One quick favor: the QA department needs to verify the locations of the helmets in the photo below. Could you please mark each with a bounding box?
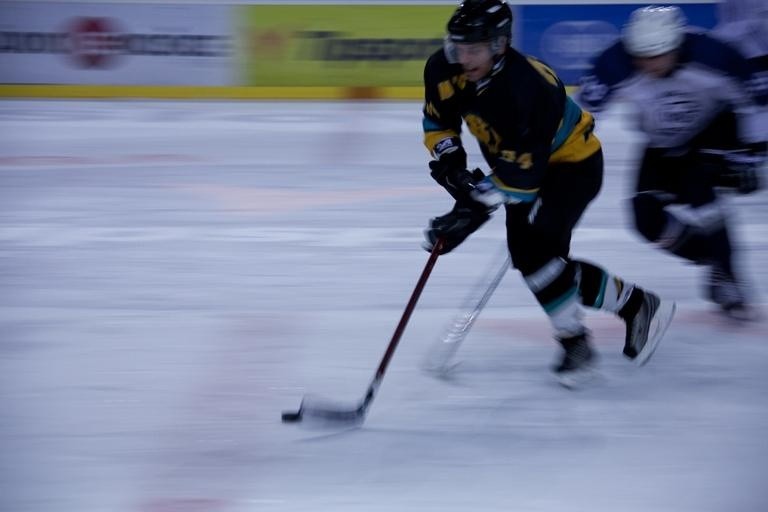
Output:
[619,6,685,58]
[447,0,513,44]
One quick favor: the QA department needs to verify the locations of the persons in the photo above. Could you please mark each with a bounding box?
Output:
[570,1,768,310]
[422,1,661,374]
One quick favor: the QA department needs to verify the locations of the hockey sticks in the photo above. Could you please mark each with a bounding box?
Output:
[430,256,512,379]
[297,238,441,430]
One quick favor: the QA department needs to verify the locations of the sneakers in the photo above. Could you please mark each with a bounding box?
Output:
[557,291,659,369]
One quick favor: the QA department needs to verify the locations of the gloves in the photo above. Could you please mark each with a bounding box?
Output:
[424,151,493,254]
